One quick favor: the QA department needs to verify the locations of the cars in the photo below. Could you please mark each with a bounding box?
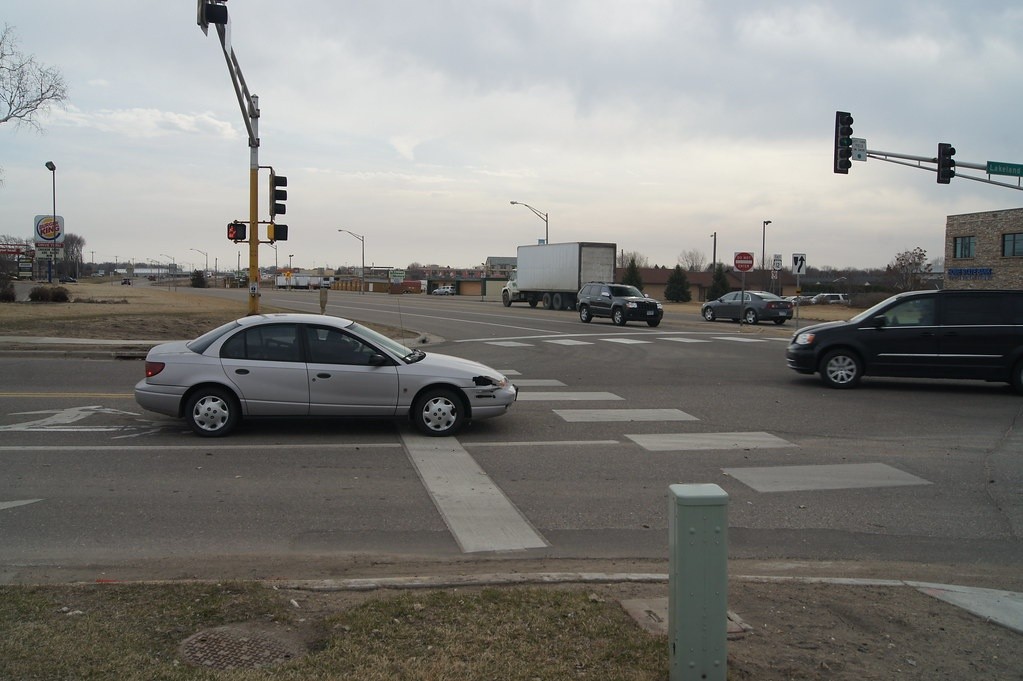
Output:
[780,294,854,308]
[121,278,131,285]
[58,276,77,283]
[134,314,519,437]
[701,290,794,325]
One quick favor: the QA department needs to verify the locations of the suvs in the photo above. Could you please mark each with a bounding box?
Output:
[576,280,663,327]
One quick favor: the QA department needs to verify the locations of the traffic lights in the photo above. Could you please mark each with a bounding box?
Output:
[226,223,246,240]
[833,111,854,175]
[936,143,956,184]
[268,174,288,216]
[196,0,229,30]
[269,224,288,241]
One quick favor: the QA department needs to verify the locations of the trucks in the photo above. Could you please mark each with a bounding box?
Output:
[500,242,616,310]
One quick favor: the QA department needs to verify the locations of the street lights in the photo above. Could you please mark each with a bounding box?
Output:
[44,160,56,278]
[190,248,208,270]
[160,254,175,272]
[510,201,550,243]
[338,230,364,293]
[146,257,161,283]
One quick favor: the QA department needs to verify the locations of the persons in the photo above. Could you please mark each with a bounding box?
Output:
[917,304,934,326]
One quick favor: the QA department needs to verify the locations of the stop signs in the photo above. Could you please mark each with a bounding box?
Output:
[734,252,755,273]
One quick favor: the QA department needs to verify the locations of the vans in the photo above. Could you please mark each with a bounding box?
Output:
[433,286,456,296]
[784,289,1023,393]
